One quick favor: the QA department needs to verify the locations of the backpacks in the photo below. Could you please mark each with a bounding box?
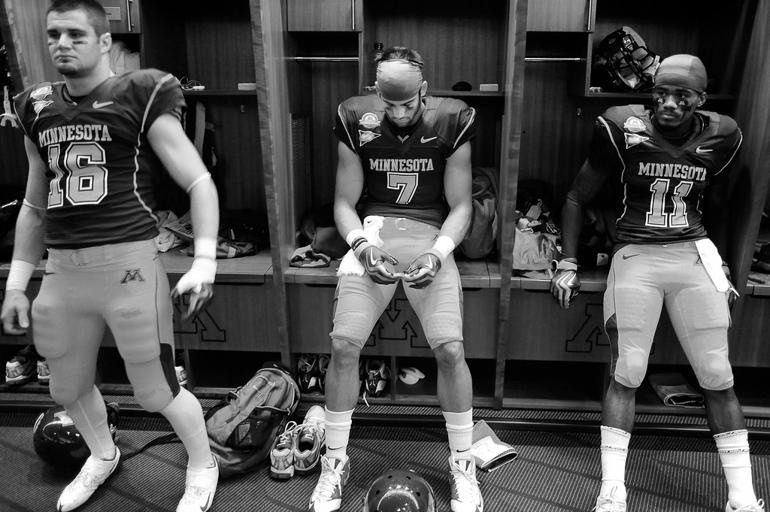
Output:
[205,366,302,476]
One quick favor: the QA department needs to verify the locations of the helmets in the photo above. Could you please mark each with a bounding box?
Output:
[364,469,437,512]
[33,399,119,469]
[597,27,660,91]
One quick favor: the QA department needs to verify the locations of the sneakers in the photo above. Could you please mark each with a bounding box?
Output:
[595,495,626,511]
[175,456,220,512]
[271,403,351,512]
[725,498,764,512]
[358,359,389,397]
[6,348,51,385]
[55,446,121,512]
[446,455,484,511]
[298,356,326,394]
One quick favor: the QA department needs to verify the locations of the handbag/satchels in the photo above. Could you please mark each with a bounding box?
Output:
[513,201,561,280]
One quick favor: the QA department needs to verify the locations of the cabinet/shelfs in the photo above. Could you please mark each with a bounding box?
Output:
[1,0,288,391]
[259,0,514,410]
[499,0,769,419]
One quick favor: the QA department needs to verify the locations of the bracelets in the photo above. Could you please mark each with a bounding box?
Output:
[3,258,35,293]
[190,237,219,260]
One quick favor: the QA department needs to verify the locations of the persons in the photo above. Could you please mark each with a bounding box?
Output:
[0,0,221,512]
[306,48,485,512]
[548,52,767,512]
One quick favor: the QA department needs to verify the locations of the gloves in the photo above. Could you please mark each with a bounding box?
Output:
[402,251,440,289]
[170,258,215,320]
[547,267,581,306]
[350,239,402,288]
